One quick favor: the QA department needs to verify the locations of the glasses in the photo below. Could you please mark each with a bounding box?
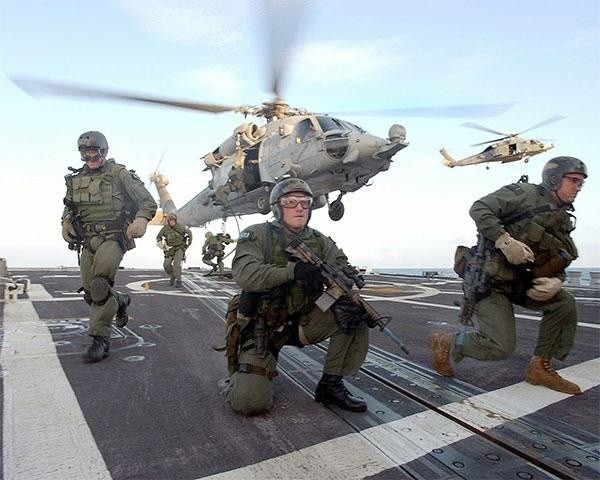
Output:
[270,195,313,209]
[563,176,585,186]
[80,148,108,161]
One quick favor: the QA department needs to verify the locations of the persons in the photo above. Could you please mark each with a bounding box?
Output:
[201,231,234,275]
[223,178,367,413]
[156,213,193,287]
[426,157,584,394]
[61,131,157,362]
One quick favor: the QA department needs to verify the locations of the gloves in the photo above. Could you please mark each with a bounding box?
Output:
[126,216,148,239]
[294,261,321,280]
[61,218,77,244]
[495,232,535,265]
[157,241,165,249]
[527,277,563,302]
[332,300,370,333]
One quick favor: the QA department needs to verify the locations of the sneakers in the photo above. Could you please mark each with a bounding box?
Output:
[116,295,131,327]
[212,264,217,271]
[86,336,109,361]
[170,278,181,287]
[219,274,224,279]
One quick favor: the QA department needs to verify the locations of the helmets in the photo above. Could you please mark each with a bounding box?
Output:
[205,232,212,238]
[270,178,313,226]
[167,213,177,225]
[78,131,108,161]
[542,156,588,190]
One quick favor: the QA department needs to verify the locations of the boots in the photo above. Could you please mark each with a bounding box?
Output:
[525,354,581,394]
[429,332,454,376]
[315,374,367,412]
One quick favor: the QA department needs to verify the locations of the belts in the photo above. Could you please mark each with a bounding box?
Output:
[84,224,122,232]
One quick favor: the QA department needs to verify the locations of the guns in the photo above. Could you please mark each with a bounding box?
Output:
[456,227,486,346]
[284,237,409,355]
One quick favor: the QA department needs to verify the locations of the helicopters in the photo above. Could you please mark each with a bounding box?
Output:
[432,111,569,175]
[9,2,521,238]
